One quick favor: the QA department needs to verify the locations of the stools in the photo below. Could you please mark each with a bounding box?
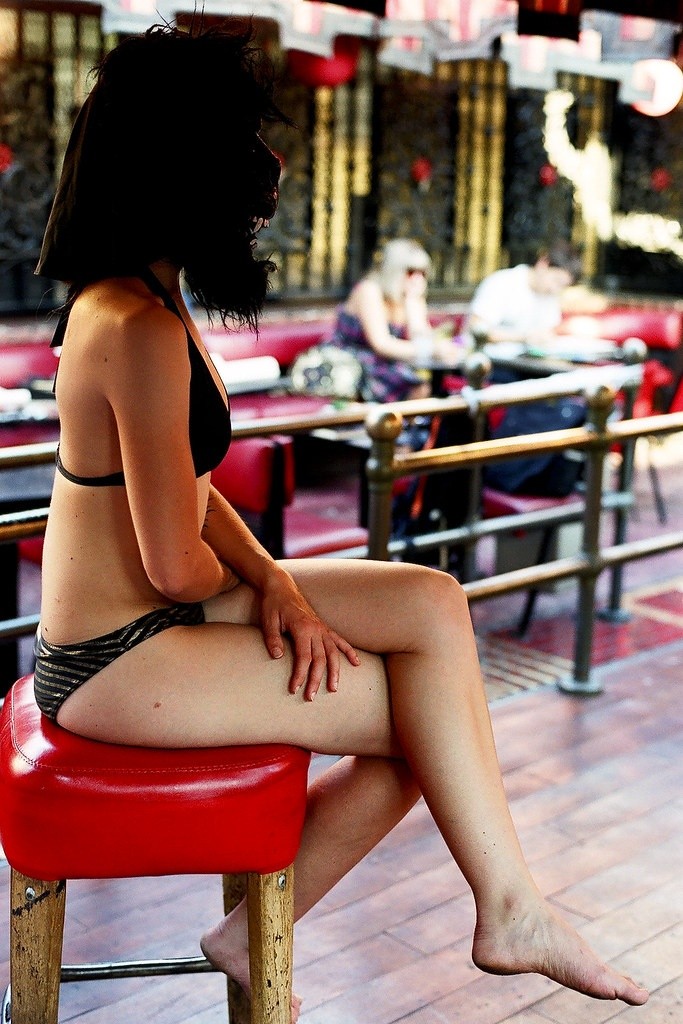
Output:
[0,672,311,1024]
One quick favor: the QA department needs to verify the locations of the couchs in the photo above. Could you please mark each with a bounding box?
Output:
[0,304,683,571]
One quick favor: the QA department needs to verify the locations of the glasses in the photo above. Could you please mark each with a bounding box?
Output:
[407,267,427,276]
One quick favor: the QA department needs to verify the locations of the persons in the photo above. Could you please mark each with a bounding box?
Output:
[31,17,649,1024]
[324,237,580,518]
